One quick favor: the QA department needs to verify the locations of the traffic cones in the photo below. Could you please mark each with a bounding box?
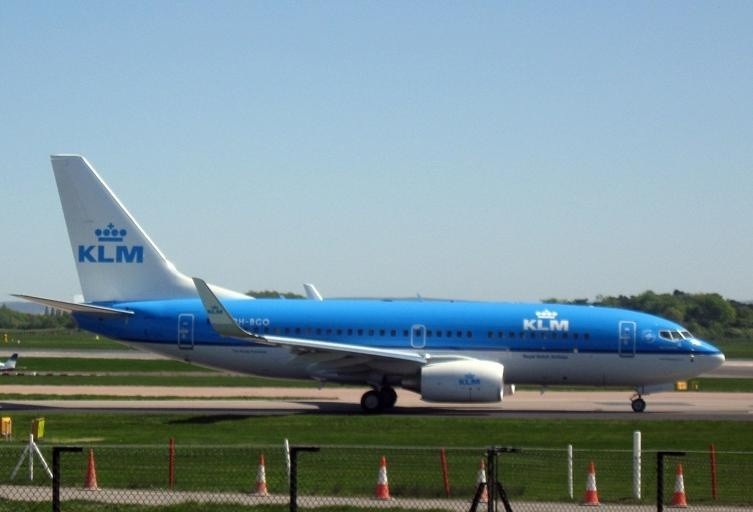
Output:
[369,454,397,501]
[667,463,689,509]
[470,458,491,503]
[77,448,104,493]
[247,455,272,499]
[580,461,602,506]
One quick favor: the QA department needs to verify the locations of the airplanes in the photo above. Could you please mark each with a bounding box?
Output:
[8,153,727,419]
[0,353,18,373]
